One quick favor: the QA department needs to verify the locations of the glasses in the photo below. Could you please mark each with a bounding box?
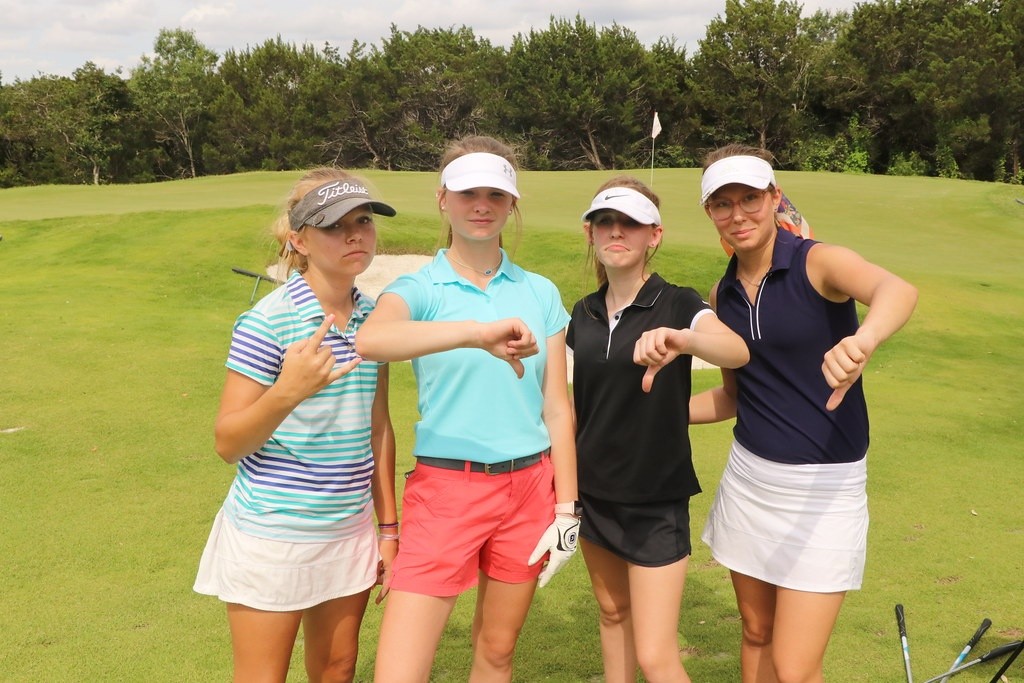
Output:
[705,188,771,220]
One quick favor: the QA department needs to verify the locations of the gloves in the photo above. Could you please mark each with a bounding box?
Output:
[528,515,581,588]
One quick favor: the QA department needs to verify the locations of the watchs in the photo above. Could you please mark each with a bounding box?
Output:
[554,501,583,517]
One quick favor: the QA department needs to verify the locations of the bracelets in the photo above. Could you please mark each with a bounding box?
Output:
[378,522,398,528]
[378,534,399,540]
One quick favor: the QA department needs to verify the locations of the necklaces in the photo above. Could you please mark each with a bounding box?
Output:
[448,254,502,275]
[742,261,771,287]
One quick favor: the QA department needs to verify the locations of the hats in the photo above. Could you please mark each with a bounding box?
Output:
[440,153,520,199]
[700,156,777,205]
[581,188,661,227]
[286,179,396,232]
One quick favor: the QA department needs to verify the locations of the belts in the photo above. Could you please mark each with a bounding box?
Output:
[417,448,550,476]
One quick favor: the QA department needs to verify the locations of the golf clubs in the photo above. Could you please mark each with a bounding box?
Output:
[895,604,1024,683]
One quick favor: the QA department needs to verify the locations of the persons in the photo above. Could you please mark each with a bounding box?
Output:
[563,180,750,683]
[689,144,919,683]
[192,171,399,683]
[355,136,579,682]
